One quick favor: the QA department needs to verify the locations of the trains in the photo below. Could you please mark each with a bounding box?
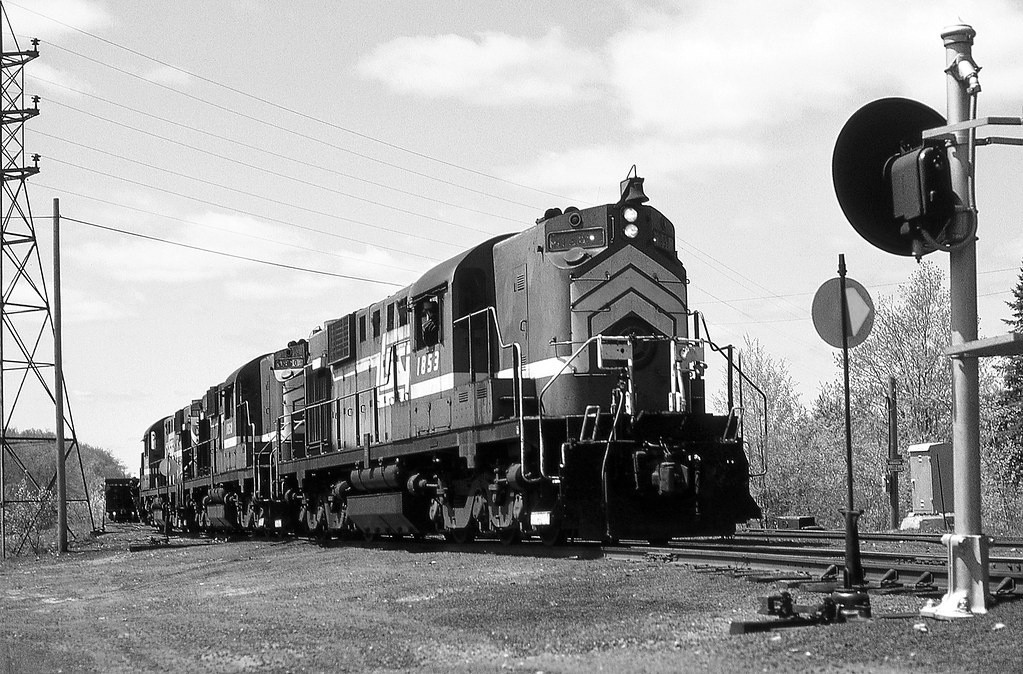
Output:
[94,166,764,543]
